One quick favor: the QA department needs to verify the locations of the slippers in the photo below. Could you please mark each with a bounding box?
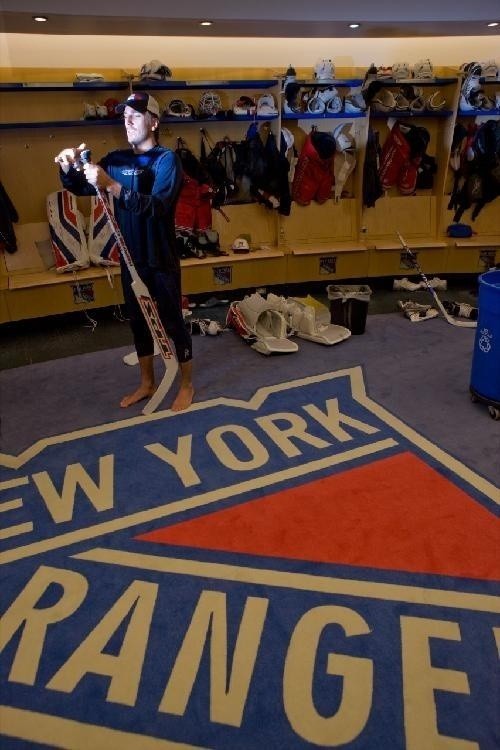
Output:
[200,297,229,307]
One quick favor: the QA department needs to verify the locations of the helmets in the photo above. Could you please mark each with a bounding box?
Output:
[140,60,171,81]
[166,99,191,117]
[473,120,500,168]
[234,95,256,116]
[199,92,223,115]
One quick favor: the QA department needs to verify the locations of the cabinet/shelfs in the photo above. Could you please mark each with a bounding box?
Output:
[1,64,500,325]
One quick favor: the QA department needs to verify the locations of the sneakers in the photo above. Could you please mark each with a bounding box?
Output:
[344,99,361,112]
[393,278,421,291]
[188,317,223,336]
[419,279,447,290]
[284,68,300,102]
[460,62,486,111]
[82,103,108,119]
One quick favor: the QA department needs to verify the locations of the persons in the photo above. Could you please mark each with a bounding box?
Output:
[54,92,194,411]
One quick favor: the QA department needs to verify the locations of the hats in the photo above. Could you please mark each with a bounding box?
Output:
[115,91,160,123]
[231,238,250,250]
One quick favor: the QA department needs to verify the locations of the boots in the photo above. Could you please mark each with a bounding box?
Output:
[443,301,473,318]
[399,300,431,316]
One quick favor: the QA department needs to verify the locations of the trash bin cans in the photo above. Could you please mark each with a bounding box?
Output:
[327,285,372,335]
[469,271,500,420]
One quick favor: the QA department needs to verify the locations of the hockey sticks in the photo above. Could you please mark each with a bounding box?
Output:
[394,228,478,328]
[78,149,180,417]
